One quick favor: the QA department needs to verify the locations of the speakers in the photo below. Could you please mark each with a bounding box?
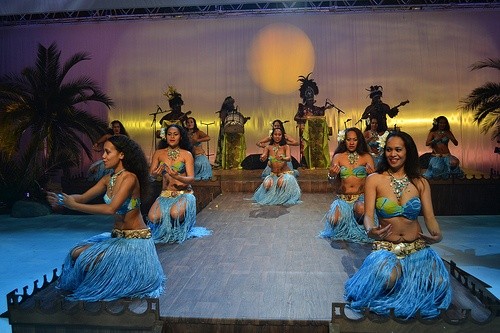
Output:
[240,154,267,170]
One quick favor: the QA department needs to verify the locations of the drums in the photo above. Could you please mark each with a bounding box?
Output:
[224,112,245,134]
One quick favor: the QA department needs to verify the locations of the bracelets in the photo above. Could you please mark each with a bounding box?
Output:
[328,173,337,179]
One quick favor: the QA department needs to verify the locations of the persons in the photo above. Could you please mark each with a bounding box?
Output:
[184,118,213,180]
[87,120,137,182]
[253,128,303,205]
[318,127,380,243]
[148,125,210,243]
[256,120,298,178]
[364,115,385,154]
[294,73,333,168]
[362,85,398,129]
[215,96,246,169]
[423,115,465,179]
[160,92,187,128]
[47,139,167,302]
[343,131,452,319]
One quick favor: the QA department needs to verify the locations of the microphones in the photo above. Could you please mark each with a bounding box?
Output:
[157,105,162,112]
[325,99,328,106]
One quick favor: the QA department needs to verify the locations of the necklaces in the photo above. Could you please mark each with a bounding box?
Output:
[388,170,409,200]
[107,169,125,190]
[273,144,282,155]
[346,150,360,165]
[168,146,179,161]
